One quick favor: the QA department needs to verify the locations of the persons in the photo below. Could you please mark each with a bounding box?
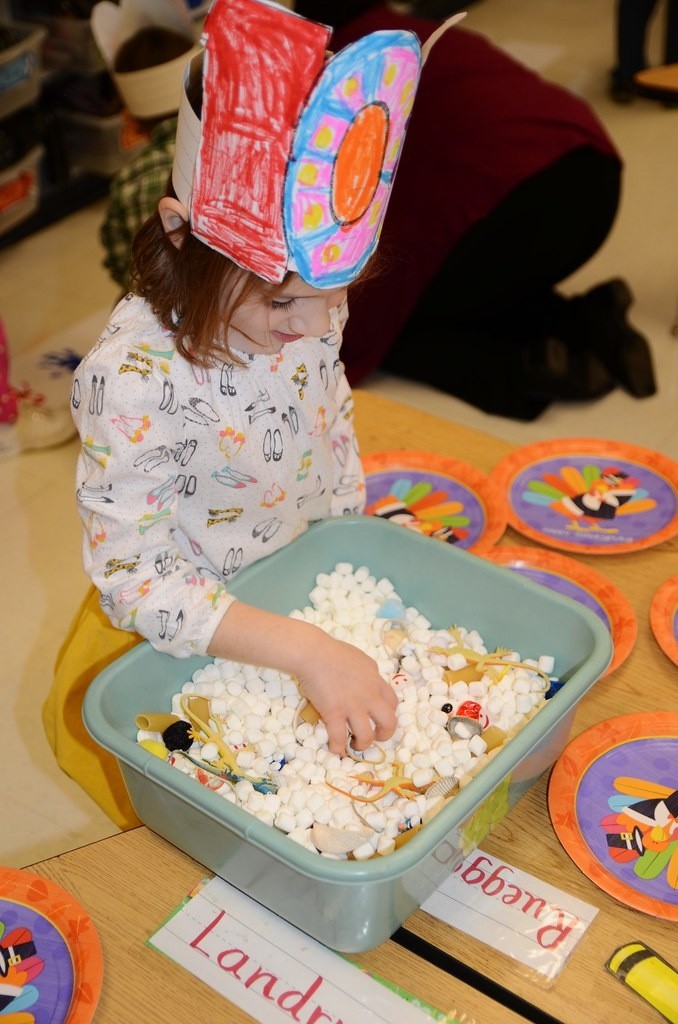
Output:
[69,0,469,759]
[89,0,678,425]
[0,316,78,456]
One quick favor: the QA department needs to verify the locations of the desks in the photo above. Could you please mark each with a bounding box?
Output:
[0,390,678,1024]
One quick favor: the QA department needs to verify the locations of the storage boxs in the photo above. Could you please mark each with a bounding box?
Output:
[0,11,153,235]
[81,516,612,951]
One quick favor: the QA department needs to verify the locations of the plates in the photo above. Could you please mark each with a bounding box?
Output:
[360,452,507,555]
[649,573,678,667]
[490,438,678,555]
[547,712,678,924]
[0,866,102,1024]
[468,546,637,682]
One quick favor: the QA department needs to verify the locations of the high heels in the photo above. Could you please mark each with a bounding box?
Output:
[518,334,617,396]
[580,278,656,398]
